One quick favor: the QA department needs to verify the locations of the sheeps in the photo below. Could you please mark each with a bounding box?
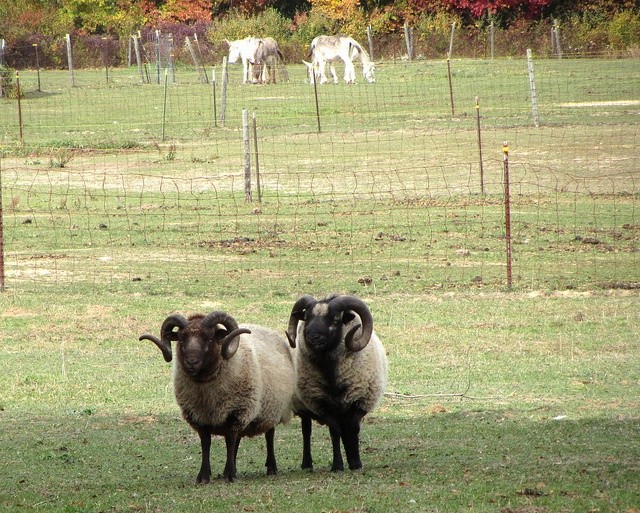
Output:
[284,295,388,475]
[138,310,296,488]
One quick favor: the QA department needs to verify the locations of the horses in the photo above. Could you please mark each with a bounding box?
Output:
[246,37,285,84]
[302,36,363,85]
[225,36,267,84]
[306,35,376,83]
